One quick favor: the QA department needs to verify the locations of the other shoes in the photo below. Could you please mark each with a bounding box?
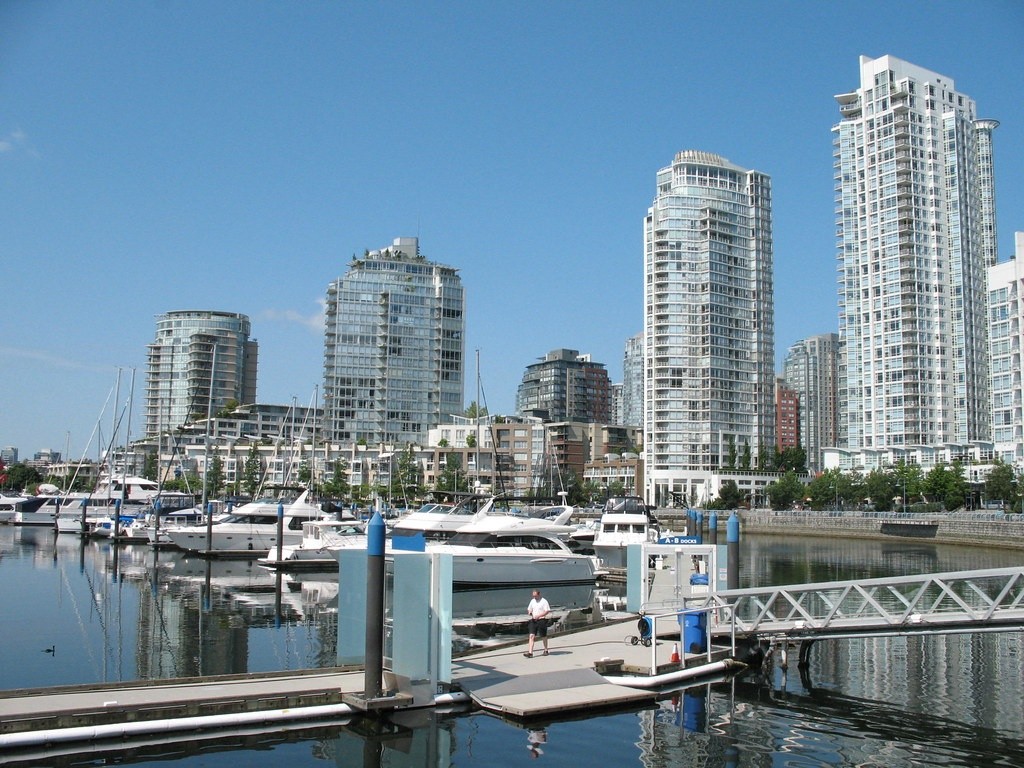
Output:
[524,653,533,657]
[541,651,549,656]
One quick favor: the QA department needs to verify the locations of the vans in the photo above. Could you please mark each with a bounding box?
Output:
[981,501,1011,511]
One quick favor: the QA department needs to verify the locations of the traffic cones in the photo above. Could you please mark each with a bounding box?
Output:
[672,643,680,662]
[670,693,677,713]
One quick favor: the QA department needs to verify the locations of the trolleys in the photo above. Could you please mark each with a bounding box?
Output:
[629,611,652,647]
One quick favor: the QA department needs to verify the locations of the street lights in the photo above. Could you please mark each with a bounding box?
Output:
[829,480,838,510]
[895,478,907,512]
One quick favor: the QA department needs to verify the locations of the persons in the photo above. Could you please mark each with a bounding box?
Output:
[523,590,550,658]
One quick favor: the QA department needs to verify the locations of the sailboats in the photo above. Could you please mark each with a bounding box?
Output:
[0,345,329,556]
[259,347,664,615]
